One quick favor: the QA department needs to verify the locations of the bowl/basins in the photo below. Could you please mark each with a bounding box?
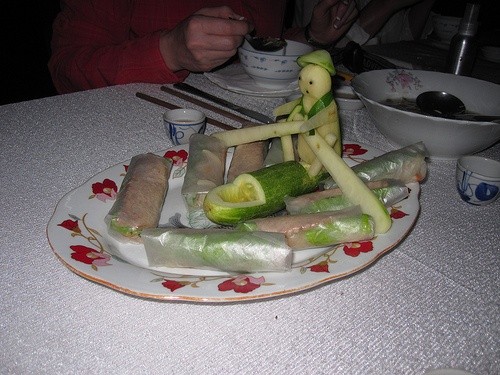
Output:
[430,8,462,45]
[238,37,314,92]
[350,69,500,160]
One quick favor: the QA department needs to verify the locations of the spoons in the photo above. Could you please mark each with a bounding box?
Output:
[227,16,288,53]
[415,90,500,122]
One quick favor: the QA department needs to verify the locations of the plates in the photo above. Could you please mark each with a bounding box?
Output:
[333,85,365,111]
[203,63,300,98]
[45,140,421,304]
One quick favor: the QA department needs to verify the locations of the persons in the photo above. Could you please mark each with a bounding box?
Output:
[47,0,438,94]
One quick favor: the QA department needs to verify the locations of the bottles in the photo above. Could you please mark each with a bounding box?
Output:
[444,0,483,76]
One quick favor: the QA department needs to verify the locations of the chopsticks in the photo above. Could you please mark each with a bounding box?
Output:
[135,85,264,131]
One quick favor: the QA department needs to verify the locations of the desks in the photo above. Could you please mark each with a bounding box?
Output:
[0,40,500,375]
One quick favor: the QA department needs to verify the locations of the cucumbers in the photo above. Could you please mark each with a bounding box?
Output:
[203,161,318,224]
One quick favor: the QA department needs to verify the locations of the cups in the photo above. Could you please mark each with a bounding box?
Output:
[162,108,206,147]
[456,154,500,207]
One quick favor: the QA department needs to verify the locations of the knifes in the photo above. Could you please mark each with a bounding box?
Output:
[172,82,275,125]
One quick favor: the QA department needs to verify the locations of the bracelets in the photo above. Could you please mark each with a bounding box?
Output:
[304,22,331,49]
